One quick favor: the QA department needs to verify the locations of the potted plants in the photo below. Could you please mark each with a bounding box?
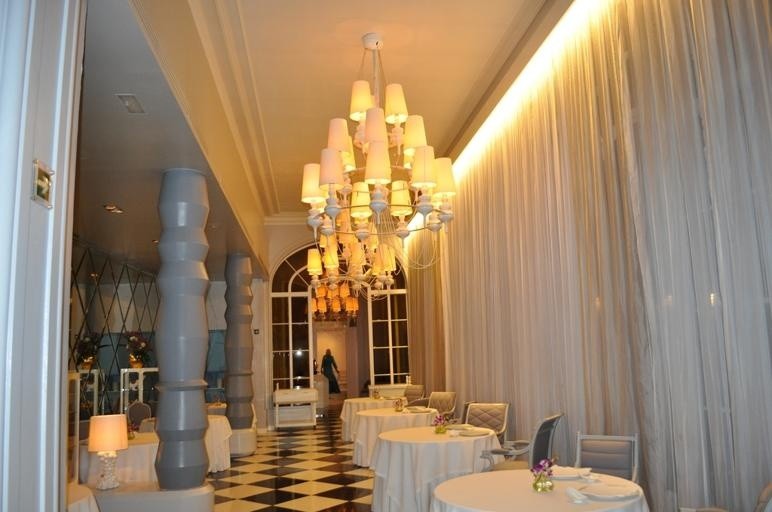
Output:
[391,398,404,412]
[123,330,153,369]
[73,334,101,371]
[530,458,555,494]
[431,415,449,434]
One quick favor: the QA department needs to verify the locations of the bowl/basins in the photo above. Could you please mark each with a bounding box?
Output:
[449,430,460,437]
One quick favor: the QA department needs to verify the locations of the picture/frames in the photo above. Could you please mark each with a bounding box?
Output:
[28,157,55,209]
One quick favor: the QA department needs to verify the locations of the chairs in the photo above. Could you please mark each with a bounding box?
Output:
[464,403,510,437]
[573,429,641,482]
[480,413,563,472]
[426,391,456,419]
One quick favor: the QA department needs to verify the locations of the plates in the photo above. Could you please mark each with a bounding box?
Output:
[460,429,490,436]
[578,486,639,501]
[445,427,467,430]
[410,410,431,412]
[550,466,593,480]
[386,397,398,400]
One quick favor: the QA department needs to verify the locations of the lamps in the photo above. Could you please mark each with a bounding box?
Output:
[87,414,130,491]
[301,40,456,240]
[308,215,397,318]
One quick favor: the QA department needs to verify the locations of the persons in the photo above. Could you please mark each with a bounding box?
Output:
[321,348,342,394]
